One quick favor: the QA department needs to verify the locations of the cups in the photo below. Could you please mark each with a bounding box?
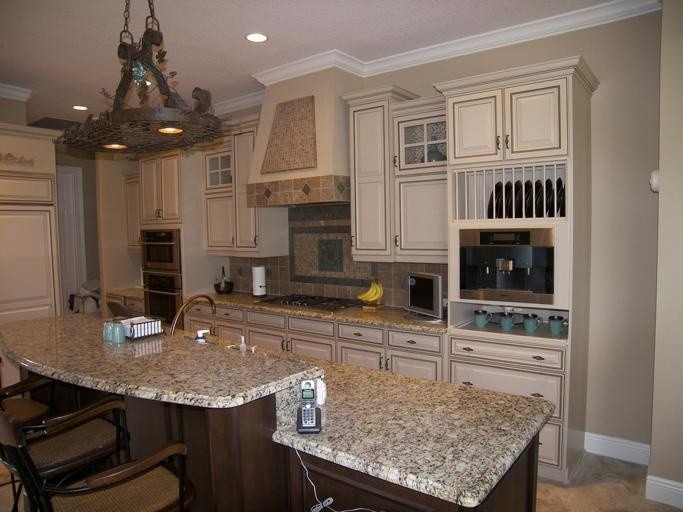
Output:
[549,315,569,336]
[103,321,113,341]
[474,310,493,328]
[112,322,125,344]
[522,313,543,333]
[498,312,519,330]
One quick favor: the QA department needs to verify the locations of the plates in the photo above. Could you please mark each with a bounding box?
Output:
[487,312,525,327]
[487,177,563,219]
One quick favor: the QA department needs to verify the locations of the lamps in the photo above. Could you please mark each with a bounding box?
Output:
[59,1,225,158]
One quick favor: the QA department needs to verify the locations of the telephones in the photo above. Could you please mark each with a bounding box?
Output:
[297,379,321,433]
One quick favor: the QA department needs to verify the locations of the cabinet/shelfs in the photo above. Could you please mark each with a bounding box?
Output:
[352,99,388,257]
[393,113,450,257]
[129,174,140,247]
[0,123,63,399]
[244,310,336,359]
[188,301,245,345]
[207,147,232,251]
[444,89,569,158]
[108,294,144,319]
[142,153,179,225]
[447,335,564,481]
[238,127,256,252]
[334,322,446,382]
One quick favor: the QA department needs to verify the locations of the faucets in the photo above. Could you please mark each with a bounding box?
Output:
[170,293,217,334]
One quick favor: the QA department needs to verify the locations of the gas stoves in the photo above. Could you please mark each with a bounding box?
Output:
[257,293,363,314]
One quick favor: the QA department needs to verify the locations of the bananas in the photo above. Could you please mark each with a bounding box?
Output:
[357,279,384,304]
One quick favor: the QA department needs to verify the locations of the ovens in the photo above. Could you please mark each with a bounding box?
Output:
[139,230,184,331]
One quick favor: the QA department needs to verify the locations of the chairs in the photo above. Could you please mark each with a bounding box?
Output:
[0,381,195,512]
